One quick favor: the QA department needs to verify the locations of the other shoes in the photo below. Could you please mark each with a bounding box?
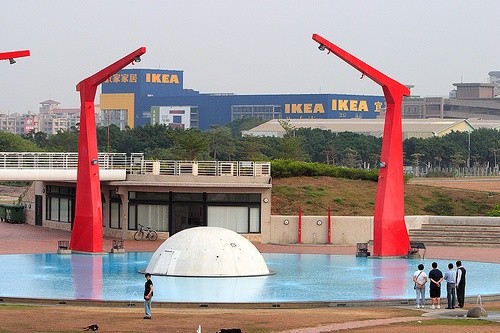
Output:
[143,316,151,319]
[457,304,463,308]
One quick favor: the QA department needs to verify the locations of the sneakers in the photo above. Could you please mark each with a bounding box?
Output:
[415,306,424,309]
[430,306,440,309]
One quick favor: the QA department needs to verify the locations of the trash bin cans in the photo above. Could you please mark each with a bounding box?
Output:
[0,203,5,223]
[6,204,24,224]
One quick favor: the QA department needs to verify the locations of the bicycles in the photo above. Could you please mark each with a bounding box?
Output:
[134,224,158,241]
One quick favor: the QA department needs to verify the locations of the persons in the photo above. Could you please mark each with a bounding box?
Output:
[455,261,466,307]
[144,272,154,320]
[413,264,428,309]
[428,262,443,309]
[443,263,456,309]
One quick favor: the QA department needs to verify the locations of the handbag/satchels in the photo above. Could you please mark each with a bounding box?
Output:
[413,283,416,289]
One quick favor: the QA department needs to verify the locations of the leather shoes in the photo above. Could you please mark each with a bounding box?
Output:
[445,306,455,309]
